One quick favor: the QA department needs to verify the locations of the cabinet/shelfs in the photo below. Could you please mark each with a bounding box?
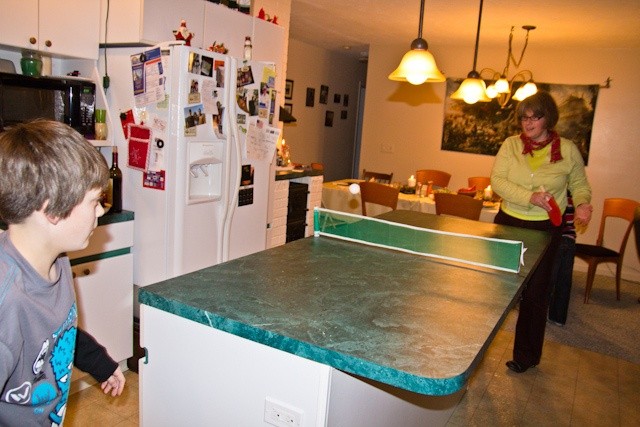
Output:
[100,0,285,71]
[266,168,324,250]
[66,220,133,395]
[3,1,112,60]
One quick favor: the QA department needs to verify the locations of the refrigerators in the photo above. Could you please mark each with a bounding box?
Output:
[104,42,278,288]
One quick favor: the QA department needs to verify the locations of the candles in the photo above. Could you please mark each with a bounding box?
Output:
[484,185,493,198]
[407,175,416,188]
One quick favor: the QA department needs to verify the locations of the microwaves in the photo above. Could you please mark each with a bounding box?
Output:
[0,71,96,140]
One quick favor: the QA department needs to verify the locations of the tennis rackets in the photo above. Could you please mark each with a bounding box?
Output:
[540,183,563,226]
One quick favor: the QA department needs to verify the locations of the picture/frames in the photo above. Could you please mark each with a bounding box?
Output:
[344,94,348,106]
[284,103,292,123]
[325,111,334,127]
[440,77,600,166]
[341,110,347,119]
[285,79,293,100]
[334,93,340,103]
[320,85,328,103]
[306,87,315,107]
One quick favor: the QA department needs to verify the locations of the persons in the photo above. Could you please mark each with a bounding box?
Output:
[238,92,247,111]
[0,118,126,427]
[213,116,218,129]
[197,109,206,124]
[216,102,221,109]
[546,188,580,328]
[212,91,218,98]
[193,82,199,95]
[260,84,270,108]
[185,109,195,127]
[242,73,250,85]
[193,112,199,125]
[489,92,592,373]
[249,96,257,116]
[217,116,222,126]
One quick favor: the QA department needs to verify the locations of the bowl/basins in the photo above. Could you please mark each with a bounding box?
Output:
[459,187,477,199]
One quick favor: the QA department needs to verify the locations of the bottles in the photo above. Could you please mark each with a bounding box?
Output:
[245,36,250,60]
[103,146,121,215]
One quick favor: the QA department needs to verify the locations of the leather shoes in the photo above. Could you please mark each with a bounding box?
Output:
[506,358,539,372]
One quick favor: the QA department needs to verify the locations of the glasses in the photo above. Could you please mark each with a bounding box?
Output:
[518,112,545,121]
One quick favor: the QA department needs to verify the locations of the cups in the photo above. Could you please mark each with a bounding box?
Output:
[95,109,107,123]
[21,49,43,78]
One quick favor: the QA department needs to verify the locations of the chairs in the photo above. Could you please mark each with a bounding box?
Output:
[575,198,640,302]
[415,169,452,188]
[468,177,491,189]
[361,168,394,184]
[359,182,398,218]
[434,192,483,221]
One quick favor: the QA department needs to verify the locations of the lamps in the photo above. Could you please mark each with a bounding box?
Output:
[450,0,492,105]
[387,0,446,86]
[480,26,538,109]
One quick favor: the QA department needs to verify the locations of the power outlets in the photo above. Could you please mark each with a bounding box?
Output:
[264,399,302,427]
